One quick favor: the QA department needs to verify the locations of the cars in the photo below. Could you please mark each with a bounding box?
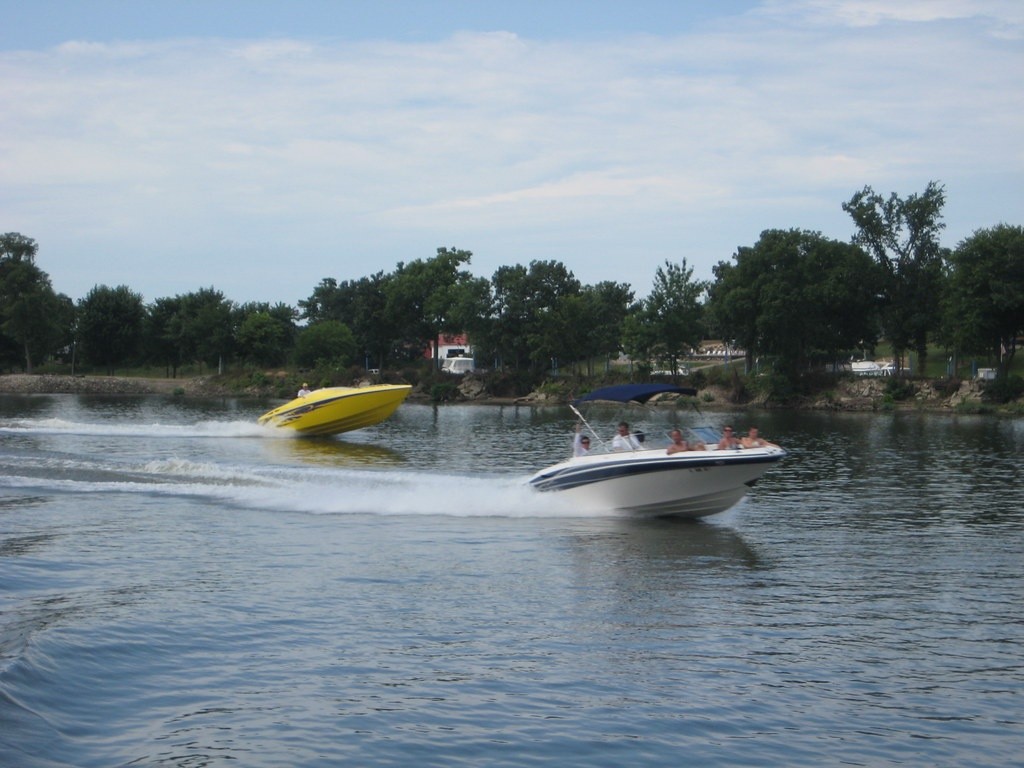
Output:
[442,357,475,374]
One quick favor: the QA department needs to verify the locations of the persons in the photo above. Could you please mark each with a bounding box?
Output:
[297,383,311,398]
[741,426,781,449]
[613,421,646,452]
[666,430,706,457]
[573,417,592,457]
[717,425,742,451]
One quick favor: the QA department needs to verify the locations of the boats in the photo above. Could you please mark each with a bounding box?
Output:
[517,382,788,524]
[257,383,413,441]
